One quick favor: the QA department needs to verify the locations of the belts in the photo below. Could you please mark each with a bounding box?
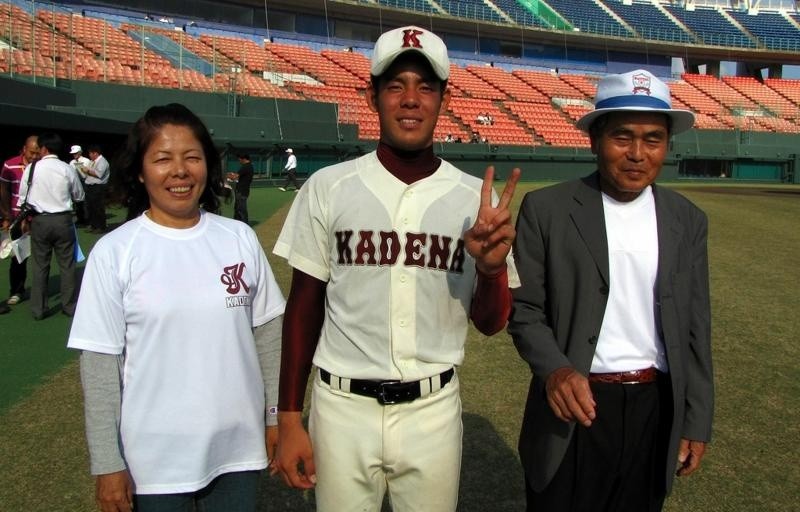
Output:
[319,368,453,405]
[589,367,662,384]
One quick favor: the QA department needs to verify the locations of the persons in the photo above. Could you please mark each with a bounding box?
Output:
[278,147,301,193]
[143,13,151,21]
[159,15,168,23]
[63,101,286,512]
[271,23,522,511]
[189,20,199,32]
[443,111,495,145]
[182,20,188,33]
[502,66,715,509]
[225,150,254,225]
[0,133,111,321]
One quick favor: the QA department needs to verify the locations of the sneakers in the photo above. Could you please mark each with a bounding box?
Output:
[9,295,20,305]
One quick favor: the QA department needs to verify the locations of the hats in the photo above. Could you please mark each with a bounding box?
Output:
[70,145,81,154]
[577,69,694,135]
[285,149,293,153]
[370,25,449,82]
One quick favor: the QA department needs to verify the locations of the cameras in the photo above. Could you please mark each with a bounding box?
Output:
[16,203,34,222]
